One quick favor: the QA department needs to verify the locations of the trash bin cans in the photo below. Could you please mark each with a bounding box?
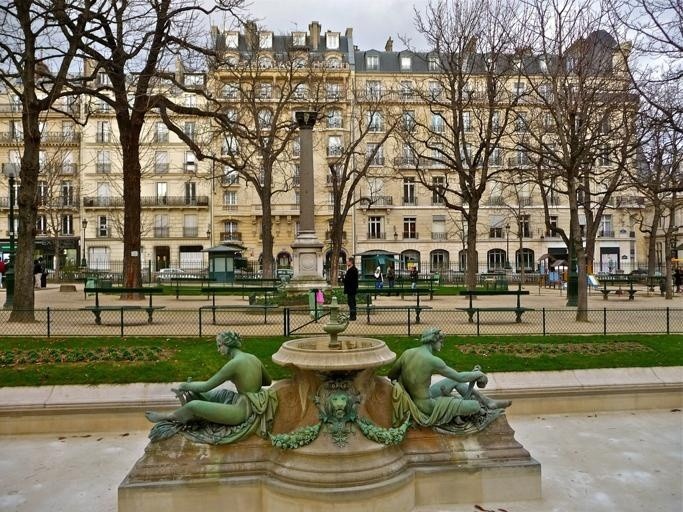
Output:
[87,277,97,296]
[434,272,442,286]
[102,280,112,294]
[484,279,508,291]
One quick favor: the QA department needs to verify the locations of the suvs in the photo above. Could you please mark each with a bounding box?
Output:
[276,269,294,285]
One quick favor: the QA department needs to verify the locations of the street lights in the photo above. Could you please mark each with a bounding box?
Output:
[503,223,513,269]
[81,218,88,266]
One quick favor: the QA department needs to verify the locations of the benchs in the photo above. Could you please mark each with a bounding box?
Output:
[599,274,676,299]
[479,274,507,287]
[166,278,281,325]
[81,283,165,328]
[352,283,535,328]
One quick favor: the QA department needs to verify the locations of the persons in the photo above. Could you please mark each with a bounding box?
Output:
[410,267,418,289]
[387,265,395,287]
[338,256,359,321]
[375,265,384,288]
[672,269,682,294]
[0,255,50,287]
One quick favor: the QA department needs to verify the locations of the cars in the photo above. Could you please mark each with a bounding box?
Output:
[628,268,665,282]
[155,268,196,283]
